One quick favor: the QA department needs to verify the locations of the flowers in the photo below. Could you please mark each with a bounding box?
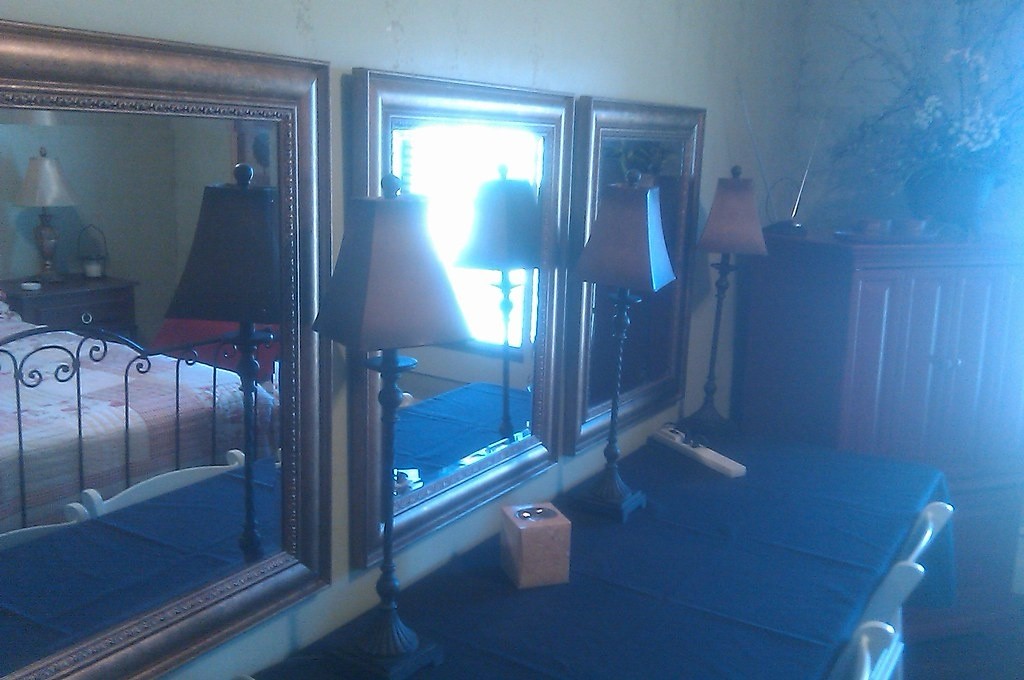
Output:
[823,0,1024,204]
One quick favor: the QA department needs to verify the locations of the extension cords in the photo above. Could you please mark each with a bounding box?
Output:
[653,427,746,478]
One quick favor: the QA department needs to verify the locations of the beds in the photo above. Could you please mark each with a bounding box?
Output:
[0,301,277,533]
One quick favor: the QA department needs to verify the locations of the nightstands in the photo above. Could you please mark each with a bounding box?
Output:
[0,271,142,344]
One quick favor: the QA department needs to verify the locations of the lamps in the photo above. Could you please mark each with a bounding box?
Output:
[574,166,679,522]
[13,145,80,285]
[311,173,474,680]
[164,162,287,566]
[457,164,543,440]
[680,163,769,441]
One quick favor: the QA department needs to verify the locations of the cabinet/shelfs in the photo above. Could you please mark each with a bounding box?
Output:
[725,230,1024,644]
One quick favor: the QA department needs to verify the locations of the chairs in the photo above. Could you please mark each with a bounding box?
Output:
[829,501,955,680]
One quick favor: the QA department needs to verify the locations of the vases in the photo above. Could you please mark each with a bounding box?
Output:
[908,172,995,239]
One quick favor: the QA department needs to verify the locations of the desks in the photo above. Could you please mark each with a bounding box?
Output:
[263,423,954,680]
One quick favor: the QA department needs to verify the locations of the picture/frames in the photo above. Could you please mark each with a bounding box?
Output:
[565,93,708,456]
[346,65,576,573]
[0,18,335,680]
